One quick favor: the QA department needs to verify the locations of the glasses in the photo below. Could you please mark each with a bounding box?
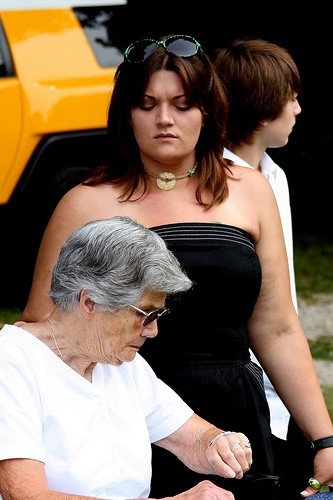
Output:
[123,35,207,73]
[127,304,170,327]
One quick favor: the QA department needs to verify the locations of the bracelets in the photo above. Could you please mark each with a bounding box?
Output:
[207,431,236,447]
[309,435,333,451]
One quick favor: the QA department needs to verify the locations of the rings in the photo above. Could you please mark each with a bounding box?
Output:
[308,477,321,489]
[241,443,252,449]
[230,441,240,451]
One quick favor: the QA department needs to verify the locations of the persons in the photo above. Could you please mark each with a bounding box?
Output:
[209,38,302,484]
[14,32,333,500]
[0,214,253,500]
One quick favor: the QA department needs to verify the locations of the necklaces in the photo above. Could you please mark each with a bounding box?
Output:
[46,318,67,364]
[141,162,198,191]
